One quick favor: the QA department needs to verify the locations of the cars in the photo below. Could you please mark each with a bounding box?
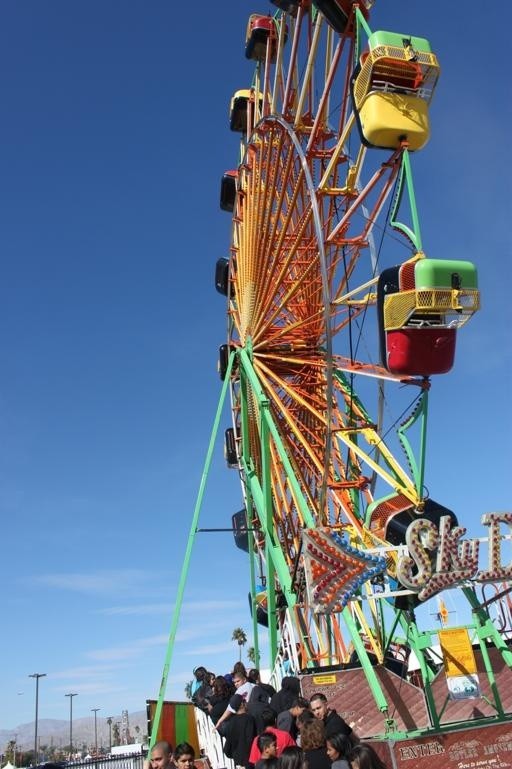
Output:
[28,752,139,769]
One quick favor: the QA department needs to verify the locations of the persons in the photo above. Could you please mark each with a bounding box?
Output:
[143,740,175,769]
[190,659,388,769]
[171,741,198,769]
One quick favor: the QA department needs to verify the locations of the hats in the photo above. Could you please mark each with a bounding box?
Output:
[229,691,248,711]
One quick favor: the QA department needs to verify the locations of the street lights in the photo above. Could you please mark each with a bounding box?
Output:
[106,716,114,753]
[116,721,123,745]
[90,708,101,759]
[29,673,48,766]
[65,692,79,763]
